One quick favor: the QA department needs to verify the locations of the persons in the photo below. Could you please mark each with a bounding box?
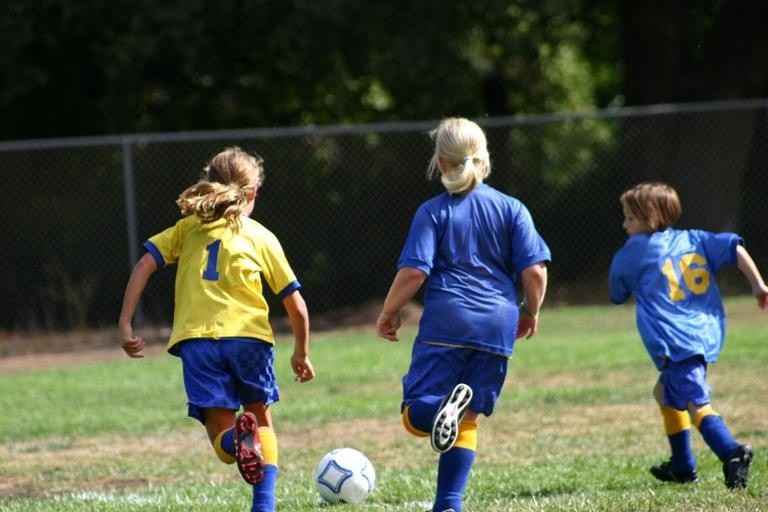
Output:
[117,143,318,511]
[374,116,552,512]
[607,181,768,490]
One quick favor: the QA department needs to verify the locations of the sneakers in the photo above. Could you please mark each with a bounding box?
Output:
[234,413,264,485]
[650,456,699,484]
[722,444,754,490]
[431,383,473,454]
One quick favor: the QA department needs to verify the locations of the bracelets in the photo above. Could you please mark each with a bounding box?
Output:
[520,303,537,317]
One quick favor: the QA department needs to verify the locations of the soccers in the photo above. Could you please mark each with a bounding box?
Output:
[315,449,376,504]
